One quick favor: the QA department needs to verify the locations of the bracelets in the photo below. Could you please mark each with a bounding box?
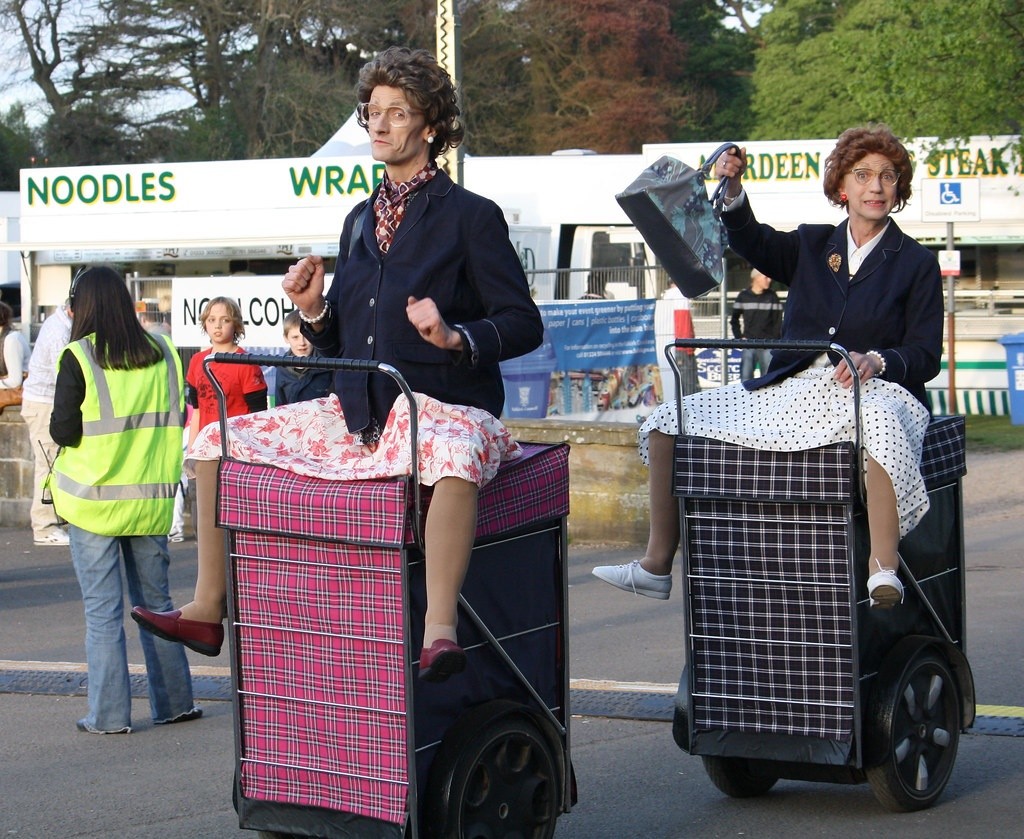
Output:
[724,196,737,201]
[299,300,329,324]
[867,351,886,377]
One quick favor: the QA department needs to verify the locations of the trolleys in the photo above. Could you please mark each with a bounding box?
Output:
[665,338,976,812]
[203,352,581,839]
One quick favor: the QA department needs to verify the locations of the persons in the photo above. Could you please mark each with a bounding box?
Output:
[729,267,782,382]
[663,279,700,397]
[275,310,333,407]
[167,397,194,543]
[0,302,31,408]
[21,304,71,545]
[41,269,203,735]
[139,295,172,338]
[130,46,544,680]
[188,295,269,454]
[591,129,943,609]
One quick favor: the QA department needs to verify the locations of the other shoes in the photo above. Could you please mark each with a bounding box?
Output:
[167,528,184,542]
[130,606,225,657]
[154,707,203,724]
[77,718,128,734]
[418,640,468,684]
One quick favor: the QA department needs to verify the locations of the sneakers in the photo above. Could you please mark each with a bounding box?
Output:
[33,528,70,546]
[592,559,673,600]
[867,558,904,610]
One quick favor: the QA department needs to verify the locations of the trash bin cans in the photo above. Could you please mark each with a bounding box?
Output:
[500,340,559,419]
[998,332,1024,425]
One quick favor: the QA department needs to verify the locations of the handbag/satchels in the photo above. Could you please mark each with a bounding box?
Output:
[615,143,742,302]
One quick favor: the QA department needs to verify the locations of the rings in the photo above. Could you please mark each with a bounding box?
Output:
[859,369,863,374]
[723,162,727,168]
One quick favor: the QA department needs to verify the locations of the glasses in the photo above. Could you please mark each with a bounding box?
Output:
[360,103,426,128]
[843,167,900,187]
